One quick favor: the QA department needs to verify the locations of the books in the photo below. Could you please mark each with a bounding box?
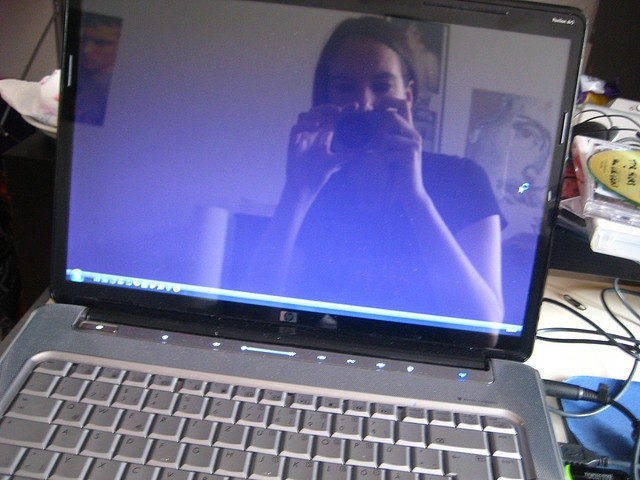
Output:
[571,135,640,262]
[557,158,587,233]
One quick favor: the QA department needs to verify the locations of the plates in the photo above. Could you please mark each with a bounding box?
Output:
[22,114,58,139]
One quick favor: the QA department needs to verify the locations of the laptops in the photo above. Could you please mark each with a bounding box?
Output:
[0,1,587,480]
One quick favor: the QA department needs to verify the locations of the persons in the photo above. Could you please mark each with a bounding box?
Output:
[406,20,441,93]
[239,17,508,350]
[75,13,122,125]
[467,91,560,243]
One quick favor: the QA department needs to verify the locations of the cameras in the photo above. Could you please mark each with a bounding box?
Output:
[331,111,390,150]
